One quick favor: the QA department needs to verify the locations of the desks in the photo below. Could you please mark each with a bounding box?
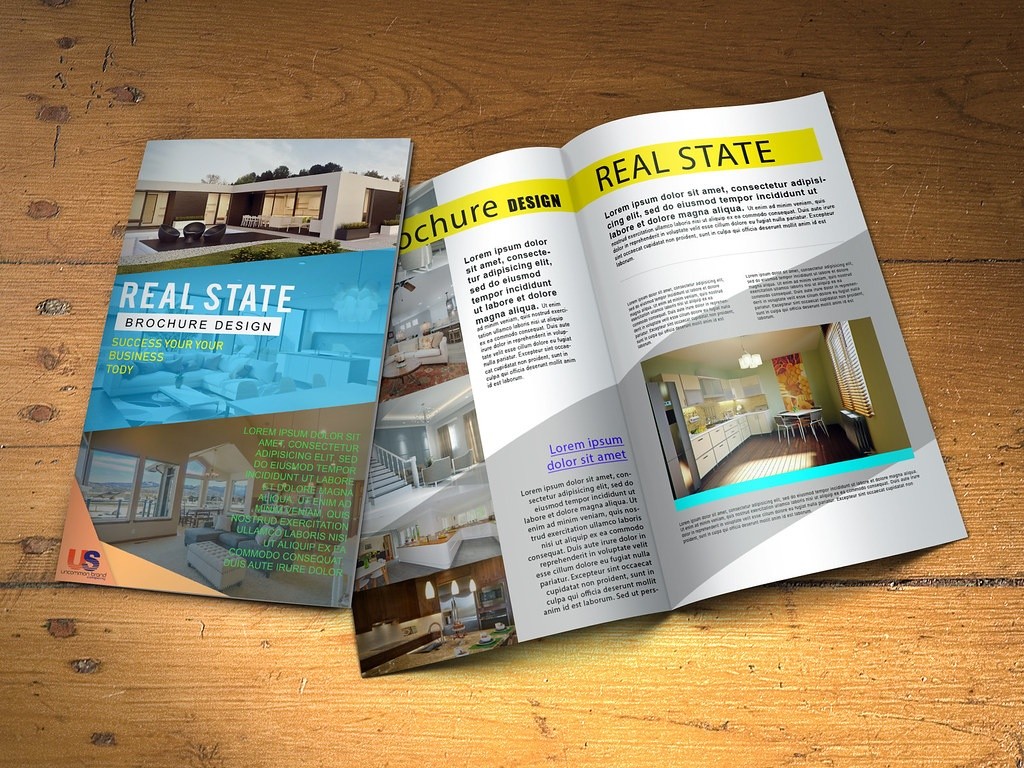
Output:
[189,507,223,527]
[781,408,822,443]
[223,382,377,417]
[355,561,388,591]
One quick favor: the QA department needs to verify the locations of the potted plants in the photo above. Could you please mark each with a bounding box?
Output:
[173,216,205,231]
[335,219,399,240]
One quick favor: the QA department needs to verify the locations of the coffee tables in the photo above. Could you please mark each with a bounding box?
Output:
[158,384,220,418]
[382,359,422,384]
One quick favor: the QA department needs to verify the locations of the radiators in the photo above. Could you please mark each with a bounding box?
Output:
[839,409,871,454]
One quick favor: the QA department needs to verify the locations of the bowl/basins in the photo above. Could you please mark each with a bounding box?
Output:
[481,635,491,641]
[496,624,505,630]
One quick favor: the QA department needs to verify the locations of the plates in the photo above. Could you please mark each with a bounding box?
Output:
[475,638,495,645]
[494,626,509,632]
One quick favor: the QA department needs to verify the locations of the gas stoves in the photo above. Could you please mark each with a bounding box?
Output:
[480,608,507,620]
[713,419,731,424]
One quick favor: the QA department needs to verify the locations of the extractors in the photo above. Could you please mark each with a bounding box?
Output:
[699,379,725,397]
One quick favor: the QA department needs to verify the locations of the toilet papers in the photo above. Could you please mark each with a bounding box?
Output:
[689,415,699,423]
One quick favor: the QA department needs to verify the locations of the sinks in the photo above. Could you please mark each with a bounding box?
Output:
[408,642,447,654]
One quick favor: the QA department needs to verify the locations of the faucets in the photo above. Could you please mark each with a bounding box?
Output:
[428,622,444,644]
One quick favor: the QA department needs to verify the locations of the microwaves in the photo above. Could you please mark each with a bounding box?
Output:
[478,583,504,608]
[684,390,704,406]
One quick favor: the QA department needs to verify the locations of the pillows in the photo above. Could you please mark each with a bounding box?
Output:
[423,332,443,349]
[214,514,284,548]
[120,353,254,376]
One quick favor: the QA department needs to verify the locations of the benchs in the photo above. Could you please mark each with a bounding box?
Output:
[187,541,247,593]
[398,448,474,487]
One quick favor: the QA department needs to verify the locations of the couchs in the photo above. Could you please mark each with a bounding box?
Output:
[183,515,295,578]
[384,331,450,366]
[103,350,278,401]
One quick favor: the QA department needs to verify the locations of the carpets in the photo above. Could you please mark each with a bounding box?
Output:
[110,388,235,427]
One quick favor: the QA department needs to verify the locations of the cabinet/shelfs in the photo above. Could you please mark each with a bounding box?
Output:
[651,373,776,479]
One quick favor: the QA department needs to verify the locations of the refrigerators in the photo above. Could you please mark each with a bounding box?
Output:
[437,575,480,636]
[646,381,703,500]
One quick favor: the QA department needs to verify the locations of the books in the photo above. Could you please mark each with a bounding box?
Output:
[55,136,414,609]
[351,91,969,678]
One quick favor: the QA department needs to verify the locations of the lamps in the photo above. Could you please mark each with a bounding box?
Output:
[425,578,476,599]
[738,335,763,370]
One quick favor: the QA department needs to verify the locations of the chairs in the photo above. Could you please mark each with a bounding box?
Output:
[158,214,323,241]
[355,557,387,591]
[774,405,830,447]
[234,373,326,416]
[179,509,204,528]
